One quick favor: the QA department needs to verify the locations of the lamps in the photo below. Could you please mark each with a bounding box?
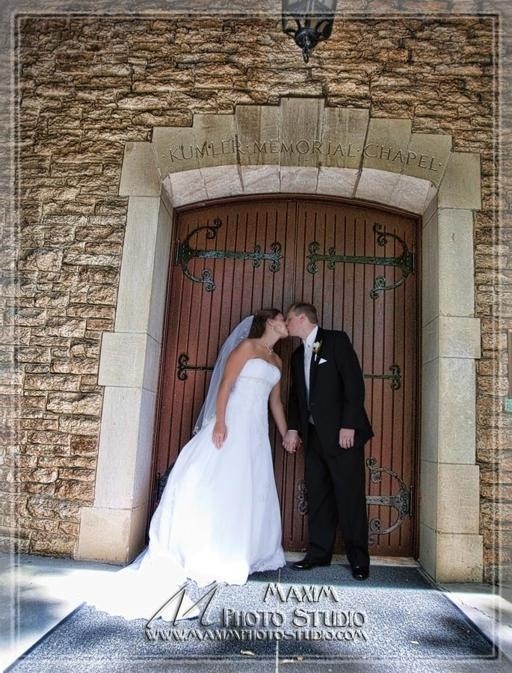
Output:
[280,0,341,66]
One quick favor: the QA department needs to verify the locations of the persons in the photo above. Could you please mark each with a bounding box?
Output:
[145,305,303,578]
[281,300,373,579]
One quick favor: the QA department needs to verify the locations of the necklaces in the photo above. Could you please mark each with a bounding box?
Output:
[264,343,273,355]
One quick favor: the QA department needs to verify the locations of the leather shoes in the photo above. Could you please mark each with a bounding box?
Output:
[351,563,371,580]
[291,553,334,571]
